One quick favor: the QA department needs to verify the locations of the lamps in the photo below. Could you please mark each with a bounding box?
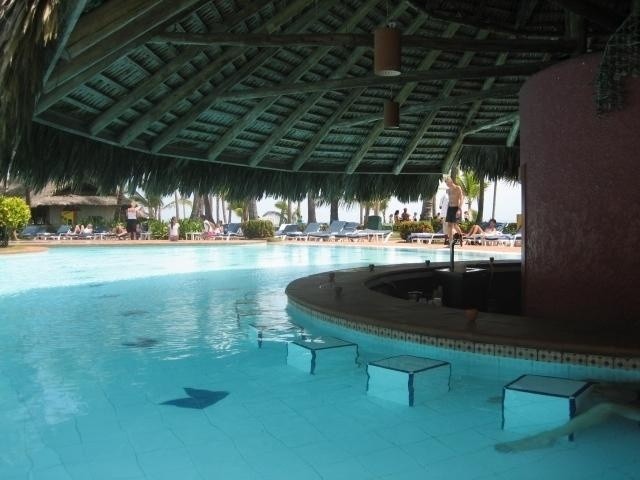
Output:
[373,0,402,130]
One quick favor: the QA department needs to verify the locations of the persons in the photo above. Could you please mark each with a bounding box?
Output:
[66,201,225,242]
[440,177,465,249]
[389,188,499,244]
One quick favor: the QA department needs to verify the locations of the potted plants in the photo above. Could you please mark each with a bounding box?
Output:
[0,194,32,247]
